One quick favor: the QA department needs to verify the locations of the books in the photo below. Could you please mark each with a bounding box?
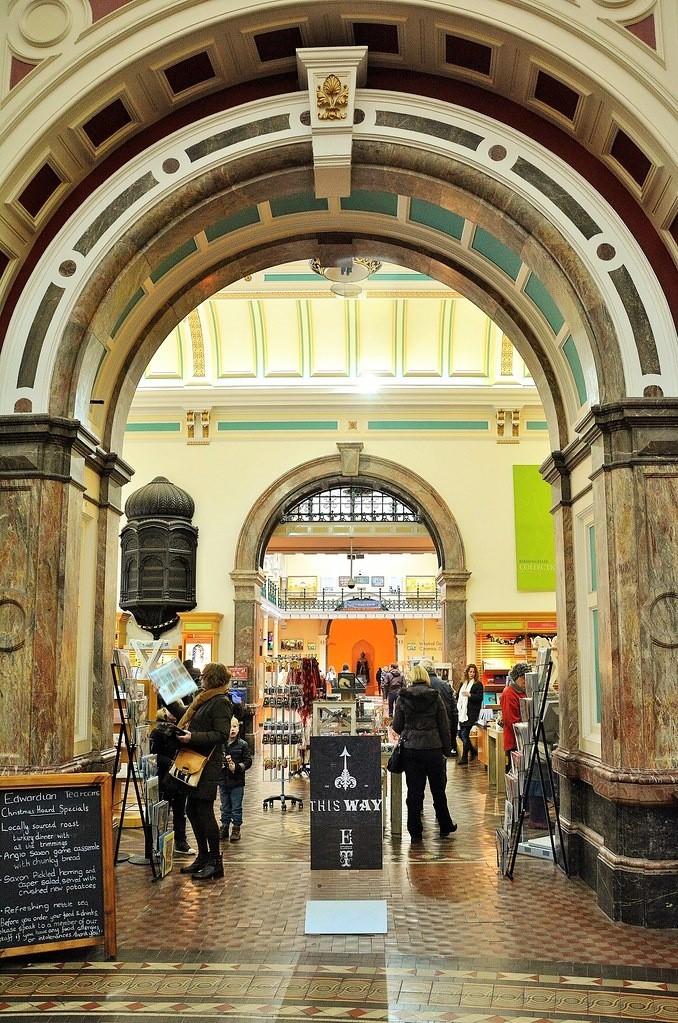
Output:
[496,647,551,876]
[115,648,176,876]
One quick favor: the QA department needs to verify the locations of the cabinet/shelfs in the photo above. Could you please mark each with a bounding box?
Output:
[110,663,172,883]
[261,655,312,813]
[501,658,569,881]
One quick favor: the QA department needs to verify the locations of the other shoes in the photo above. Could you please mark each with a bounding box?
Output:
[389,716,393,719]
[457,758,468,765]
[440,823,457,837]
[528,819,555,829]
[411,833,422,843]
[470,750,478,761]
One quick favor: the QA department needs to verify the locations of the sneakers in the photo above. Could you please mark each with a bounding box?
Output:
[174,842,196,854]
[230,826,241,840]
[219,825,229,839]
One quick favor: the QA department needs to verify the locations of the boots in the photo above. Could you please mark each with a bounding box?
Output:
[192,853,224,878]
[180,854,208,873]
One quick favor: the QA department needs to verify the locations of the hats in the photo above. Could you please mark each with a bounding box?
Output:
[509,663,532,681]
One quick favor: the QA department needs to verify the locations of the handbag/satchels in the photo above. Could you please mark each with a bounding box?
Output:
[386,744,405,774]
[169,748,207,787]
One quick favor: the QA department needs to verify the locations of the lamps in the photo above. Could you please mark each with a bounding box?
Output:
[309,257,383,282]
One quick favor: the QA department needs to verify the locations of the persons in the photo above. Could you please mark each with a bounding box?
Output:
[355,652,370,684]
[500,663,532,774]
[148,661,252,880]
[376,661,484,844]
[324,666,336,680]
[341,665,349,673]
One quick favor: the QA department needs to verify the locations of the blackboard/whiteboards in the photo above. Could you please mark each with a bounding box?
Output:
[0,772,117,959]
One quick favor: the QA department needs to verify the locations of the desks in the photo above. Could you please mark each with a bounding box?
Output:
[313,700,403,837]
